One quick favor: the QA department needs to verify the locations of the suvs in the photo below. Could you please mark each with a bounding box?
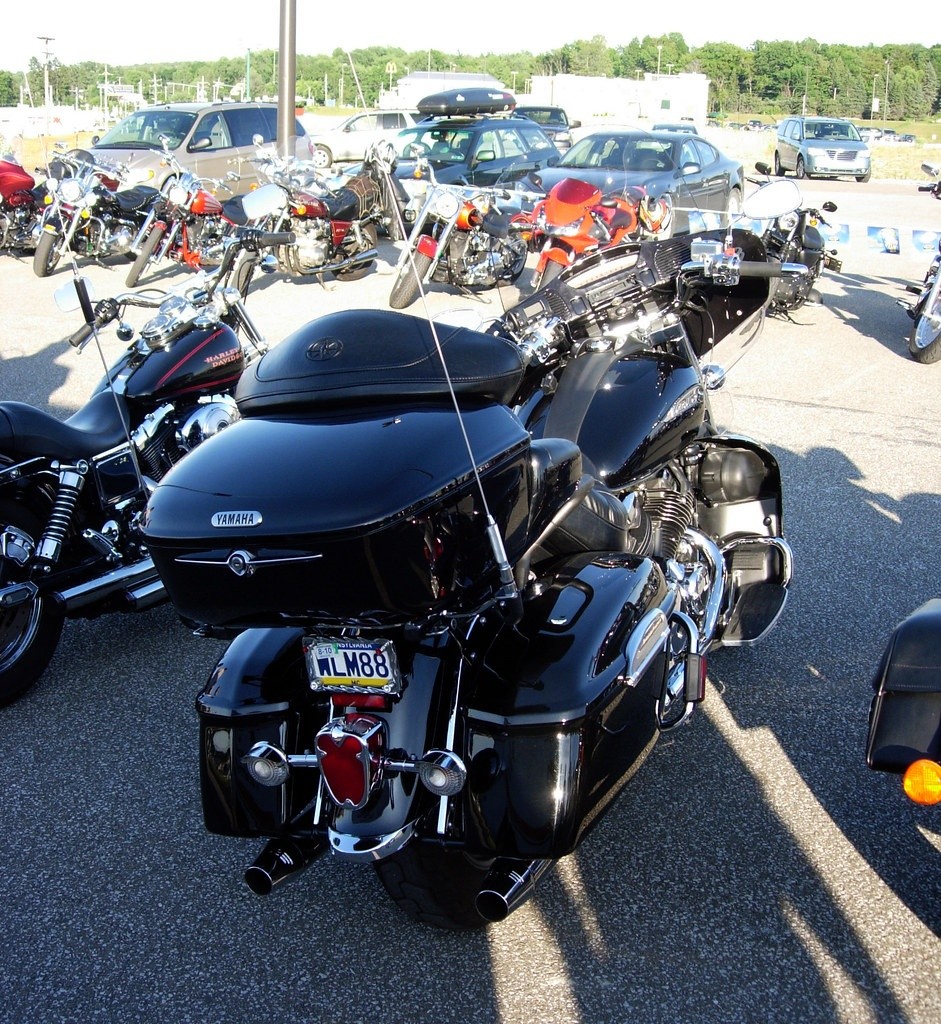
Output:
[88,99,318,223]
[340,86,563,239]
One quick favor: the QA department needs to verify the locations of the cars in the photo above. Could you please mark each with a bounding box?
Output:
[305,109,487,171]
[653,123,698,136]
[511,104,581,164]
[514,128,745,255]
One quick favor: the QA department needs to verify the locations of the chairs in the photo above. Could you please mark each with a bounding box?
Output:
[456,138,473,156]
[200,119,223,150]
[502,139,523,158]
[825,127,842,138]
[805,124,816,137]
[602,148,669,170]
[430,141,449,155]
[477,133,493,155]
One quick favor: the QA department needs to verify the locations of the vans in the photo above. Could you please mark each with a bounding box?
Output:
[775,115,872,182]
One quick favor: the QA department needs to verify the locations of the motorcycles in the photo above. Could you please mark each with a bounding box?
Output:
[133,179,812,929]
[894,163,941,365]
[1,183,290,715]
[1,137,843,309]
